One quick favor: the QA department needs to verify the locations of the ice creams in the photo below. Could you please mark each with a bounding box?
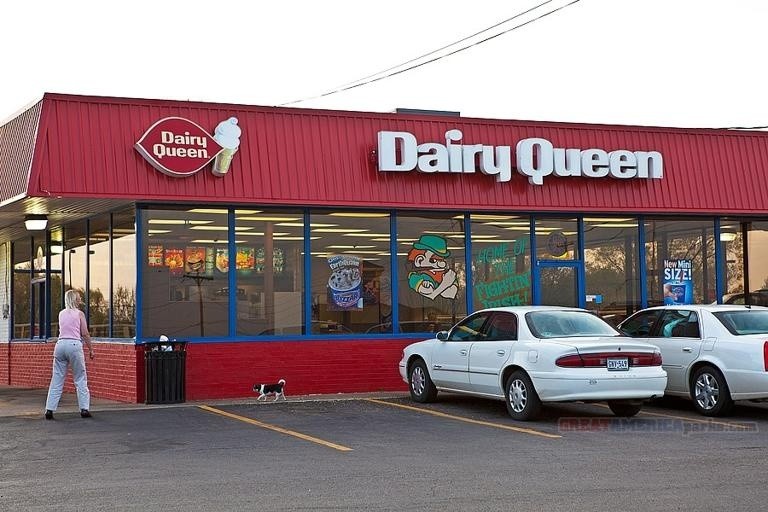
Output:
[211,117,241,178]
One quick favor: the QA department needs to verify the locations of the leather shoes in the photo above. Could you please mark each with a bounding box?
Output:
[45,410,54,420]
[80,410,92,418]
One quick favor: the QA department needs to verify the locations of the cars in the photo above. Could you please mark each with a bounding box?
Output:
[365,321,455,334]
[618,289,768,418]
[257,320,354,336]
[399,305,668,422]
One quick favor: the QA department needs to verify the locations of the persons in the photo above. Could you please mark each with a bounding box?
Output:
[663,283,677,302]
[44,289,95,420]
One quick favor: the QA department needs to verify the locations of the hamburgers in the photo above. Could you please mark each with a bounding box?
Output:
[187,253,202,270]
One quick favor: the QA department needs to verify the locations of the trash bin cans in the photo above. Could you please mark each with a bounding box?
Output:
[142,341,187,405]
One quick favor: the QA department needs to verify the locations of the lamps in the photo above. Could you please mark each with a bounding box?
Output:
[50,240,62,255]
[23,213,49,231]
[720,228,737,242]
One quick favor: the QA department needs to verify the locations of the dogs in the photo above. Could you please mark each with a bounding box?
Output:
[251,378,289,402]
